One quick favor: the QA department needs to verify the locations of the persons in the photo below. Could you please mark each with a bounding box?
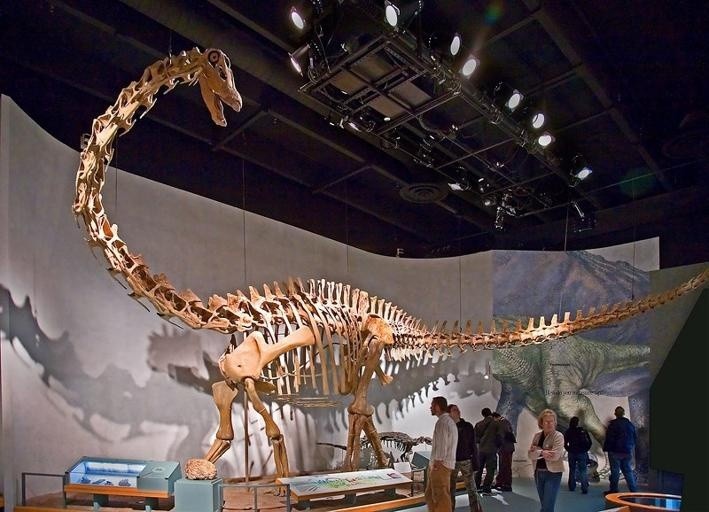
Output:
[473,407,505,497]
[447,404,485,512]
[562,416,592,495]
[425,397,459,512]
[604,405,639,503]
[527,409,564,512]
[492,412,516,491]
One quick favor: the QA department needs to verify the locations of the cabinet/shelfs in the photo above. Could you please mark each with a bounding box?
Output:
[64,456,182,512]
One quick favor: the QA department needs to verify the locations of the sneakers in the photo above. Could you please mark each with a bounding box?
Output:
[482,491,497,496]
